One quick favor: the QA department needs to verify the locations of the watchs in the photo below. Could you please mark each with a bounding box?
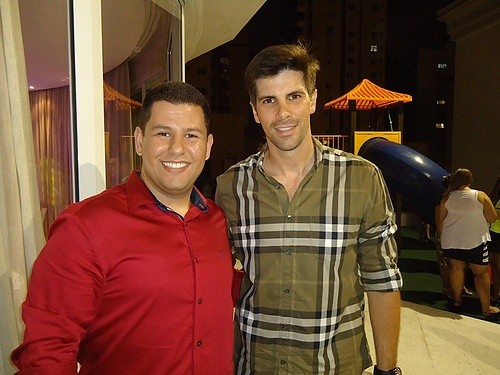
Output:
[373,365,402,375]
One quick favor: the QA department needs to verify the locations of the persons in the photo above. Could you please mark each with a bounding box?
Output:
[435,168,500,313]
[215,44,403,375]
[11,81,244,375]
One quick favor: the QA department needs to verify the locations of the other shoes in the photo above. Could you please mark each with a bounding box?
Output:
[454,301,462,307]
[483,307,500,314]
[442,287,452,298]
[462,285,473,296]
